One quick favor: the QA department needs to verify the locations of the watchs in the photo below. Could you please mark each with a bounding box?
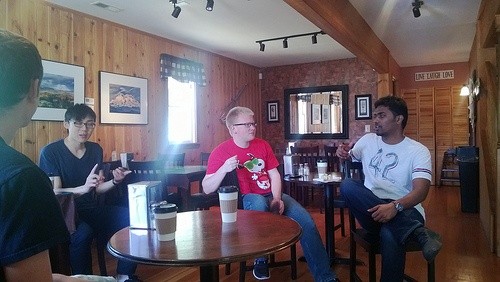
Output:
[391,200,403,213]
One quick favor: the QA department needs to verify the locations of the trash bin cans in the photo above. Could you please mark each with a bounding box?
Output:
[457,146,480,214]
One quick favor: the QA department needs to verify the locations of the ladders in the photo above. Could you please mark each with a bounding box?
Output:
[439,151,461,185]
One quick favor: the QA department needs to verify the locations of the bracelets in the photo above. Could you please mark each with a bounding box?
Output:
[112,178,118,185]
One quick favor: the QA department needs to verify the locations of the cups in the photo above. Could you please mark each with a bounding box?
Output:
[316,160,328,177]
[152,203,178,242]
[218,185,239,223]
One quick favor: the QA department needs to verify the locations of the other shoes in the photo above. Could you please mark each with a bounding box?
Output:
[415,227,443,263]
[253,258,271,280]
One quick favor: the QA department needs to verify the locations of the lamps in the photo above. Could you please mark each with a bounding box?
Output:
[412,1,424,17]
[169,0,181,18]
[206,0,215,11]
[258,42,265,51]
[312,34,317,44]
[283,38,288,48]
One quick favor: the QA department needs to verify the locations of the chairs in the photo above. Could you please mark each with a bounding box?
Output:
[99,144,434,282]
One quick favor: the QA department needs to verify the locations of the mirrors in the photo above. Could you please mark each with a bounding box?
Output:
[284,84,349,139]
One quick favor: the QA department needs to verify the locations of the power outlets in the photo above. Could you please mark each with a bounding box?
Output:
[365,124,370,133]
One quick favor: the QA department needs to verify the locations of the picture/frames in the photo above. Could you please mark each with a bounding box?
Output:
[311,103,322,125]
[30,59,86,122]
[322,104,331,123]
[355,94,372,120]
[267,100,280,123]
[98,69,149,126]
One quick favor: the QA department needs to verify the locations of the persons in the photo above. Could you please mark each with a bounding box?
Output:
[202,107,341,282]
[38,103,140,282]
[336,95,443,282]
[0,30,90,282]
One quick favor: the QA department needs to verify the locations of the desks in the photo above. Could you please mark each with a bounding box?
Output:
[283,171,362,268]
[107,209,302,282]
[134,165,209,212]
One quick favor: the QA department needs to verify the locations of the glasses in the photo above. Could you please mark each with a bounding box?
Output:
[233,122,258,128]
[70,119,96,129]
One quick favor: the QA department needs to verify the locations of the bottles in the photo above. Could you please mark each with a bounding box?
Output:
[298,164,303,176]
[324,172,331,181]
[304,163,310,176]
